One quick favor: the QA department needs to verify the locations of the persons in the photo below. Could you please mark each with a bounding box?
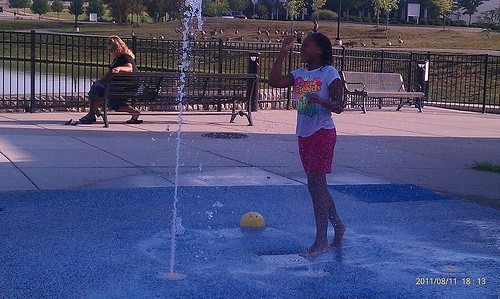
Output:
[76,35,144,125]
[309,20,319,34]
[269,33,346,256]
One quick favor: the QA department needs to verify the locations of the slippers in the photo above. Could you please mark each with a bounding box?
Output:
[126,118,143,124]
[80,116,96,124]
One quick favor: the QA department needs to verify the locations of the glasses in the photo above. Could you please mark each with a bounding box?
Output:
[109,48,114,53]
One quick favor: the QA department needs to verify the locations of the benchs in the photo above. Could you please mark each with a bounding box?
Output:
[88,71,258,128]
[340,71,425,114]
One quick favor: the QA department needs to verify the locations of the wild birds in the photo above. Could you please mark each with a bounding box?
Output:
[130,25,404,47]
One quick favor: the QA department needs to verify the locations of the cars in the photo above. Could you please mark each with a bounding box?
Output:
[219,12,234,19]
[231,11,248,19]
